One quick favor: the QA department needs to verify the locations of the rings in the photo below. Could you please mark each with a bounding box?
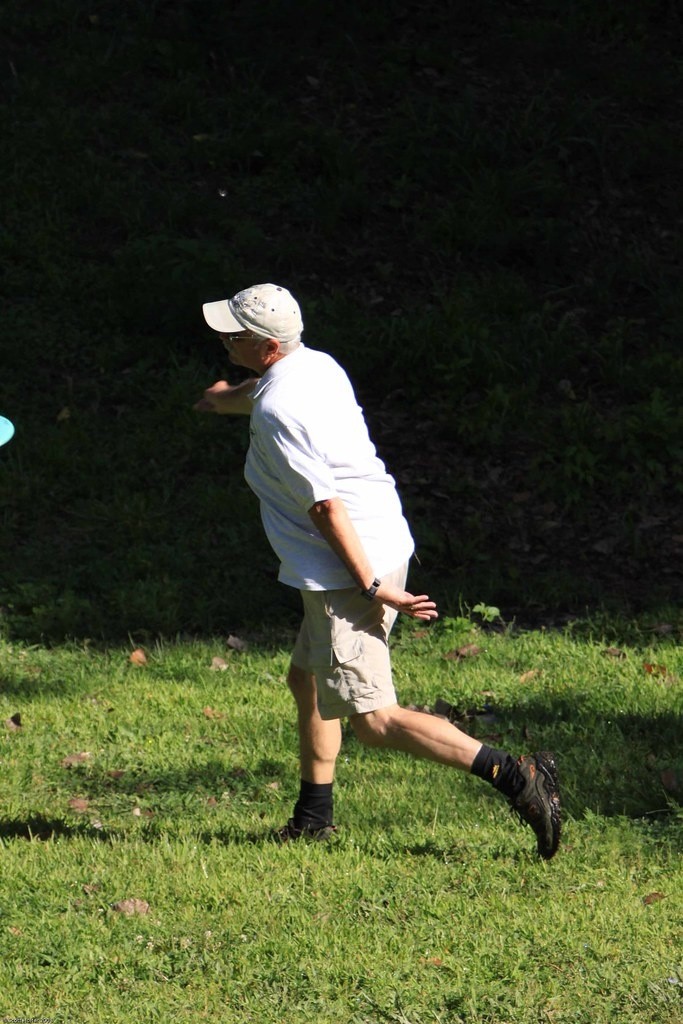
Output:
[409,605,412,611]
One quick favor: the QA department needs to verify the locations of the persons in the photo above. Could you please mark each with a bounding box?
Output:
[194,283,563,862]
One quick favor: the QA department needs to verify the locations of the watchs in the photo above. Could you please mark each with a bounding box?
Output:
[360,578,382,602]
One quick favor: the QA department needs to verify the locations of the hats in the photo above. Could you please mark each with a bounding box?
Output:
[202,283,303,347]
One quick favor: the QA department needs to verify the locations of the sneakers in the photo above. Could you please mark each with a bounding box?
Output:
[273,817,337,846]
[508,751,561,859]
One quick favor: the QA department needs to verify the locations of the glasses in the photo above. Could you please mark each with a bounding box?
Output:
[224,333,254,342]
